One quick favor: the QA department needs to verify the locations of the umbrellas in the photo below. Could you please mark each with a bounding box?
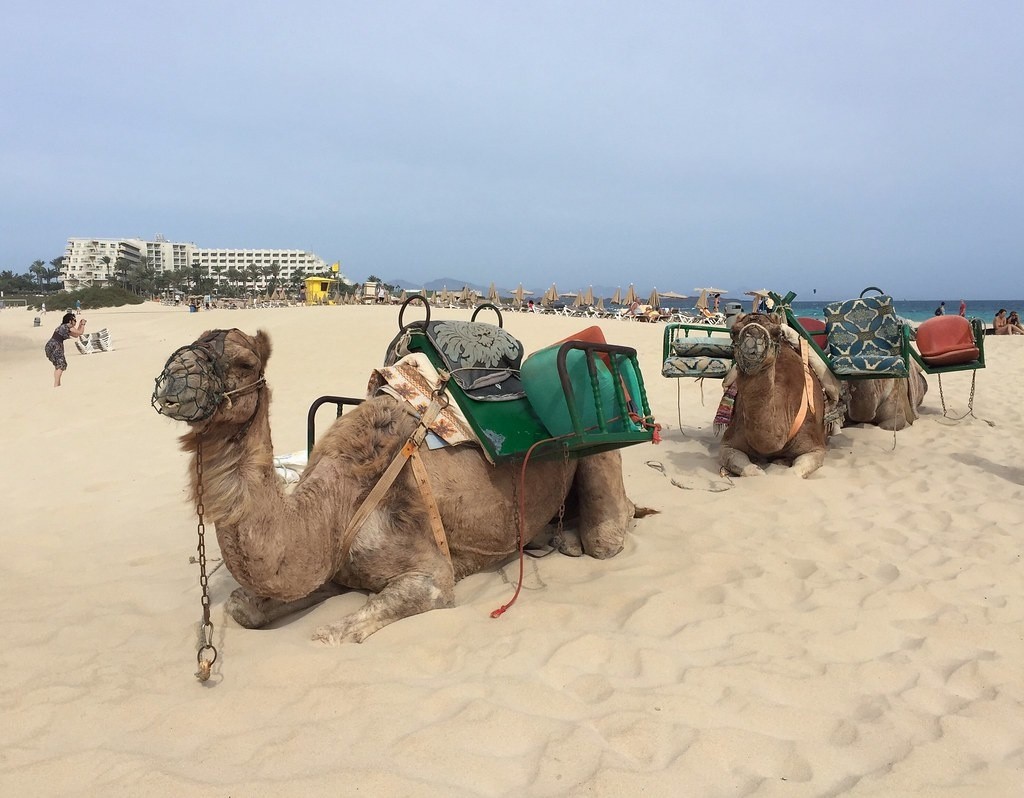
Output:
[248,281,782,326]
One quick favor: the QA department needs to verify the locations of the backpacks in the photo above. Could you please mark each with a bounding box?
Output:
[935,307,942,316]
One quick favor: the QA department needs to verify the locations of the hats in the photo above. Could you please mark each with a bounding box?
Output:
[942,302,945,305]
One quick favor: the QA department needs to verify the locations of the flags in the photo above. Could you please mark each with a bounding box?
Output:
[331,263,338,271]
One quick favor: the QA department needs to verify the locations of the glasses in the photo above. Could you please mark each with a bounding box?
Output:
[72,319,76,322]
[1011,314,1017,316]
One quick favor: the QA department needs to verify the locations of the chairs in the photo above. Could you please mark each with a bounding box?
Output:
[916,314,986,374]
[160,297,362,310]
[501,327,655,462]
[362,293,726,328]
[662,323,735,378]
[797,293,910,378]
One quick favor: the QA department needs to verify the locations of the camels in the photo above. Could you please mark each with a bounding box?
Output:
[149,326,659,643]
[716,305,928,479]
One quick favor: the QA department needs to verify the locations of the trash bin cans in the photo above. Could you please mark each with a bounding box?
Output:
[725,303,742,328]
[34,317,41,327]
[189,305,196,313]
[67,308,72,314]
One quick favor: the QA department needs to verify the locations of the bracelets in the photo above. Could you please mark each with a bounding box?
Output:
[80,323,85,326]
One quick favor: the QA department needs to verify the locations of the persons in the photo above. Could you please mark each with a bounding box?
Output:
[756,295,773,313]
[76,298,82,316]
[935,302,946,317]
[377,286,385,304]
[188,299,238,311]
[994,309,1024,335]
[45,313,87,388]
[960,299,966,316]
[174,293,180,307]
